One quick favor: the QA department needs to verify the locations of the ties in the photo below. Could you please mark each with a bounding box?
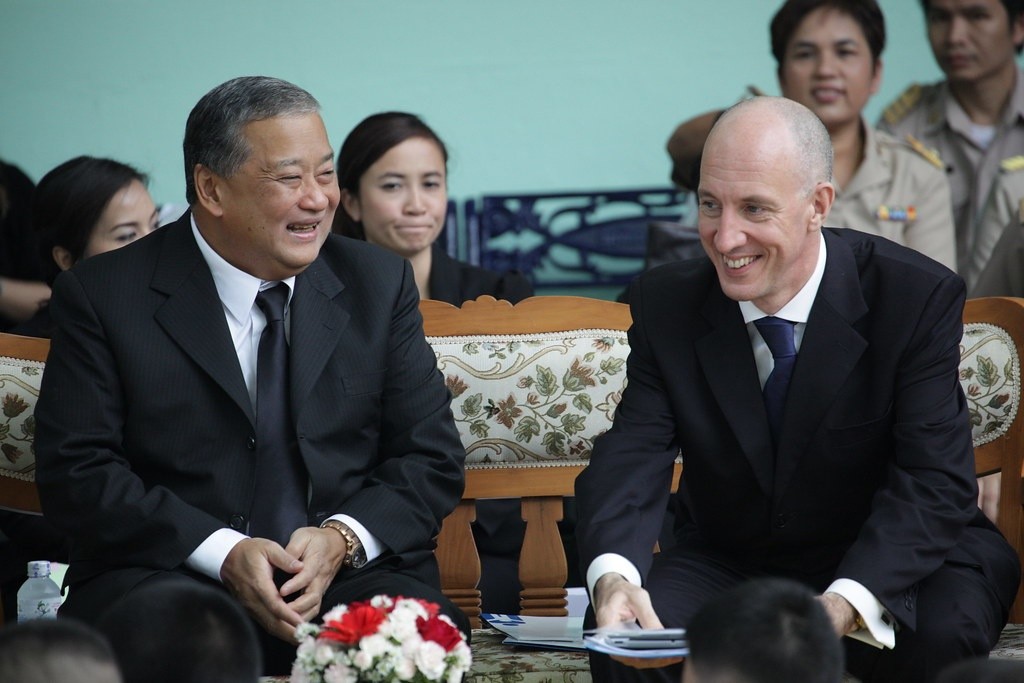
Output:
[753,316,796,426]
[255,283,308,597]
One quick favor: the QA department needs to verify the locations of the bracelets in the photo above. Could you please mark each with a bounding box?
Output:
[855,612,863,631]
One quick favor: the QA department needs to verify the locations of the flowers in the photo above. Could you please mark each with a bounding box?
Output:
[289,591,473,683]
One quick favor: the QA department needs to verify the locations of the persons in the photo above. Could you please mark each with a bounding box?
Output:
[876,0,1024,299]
[565,94,1021,683]
[327,112,535,307]
[1,155,166,338]
[96,581,263,683]
[768,0,958,274]
[683,577,845,683]
[0,616,121,683]
[29,75,474,678]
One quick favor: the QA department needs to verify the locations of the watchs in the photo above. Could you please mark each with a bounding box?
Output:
[323,520,366,571]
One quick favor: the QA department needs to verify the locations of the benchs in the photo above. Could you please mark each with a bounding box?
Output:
[1,289,1024,683]
